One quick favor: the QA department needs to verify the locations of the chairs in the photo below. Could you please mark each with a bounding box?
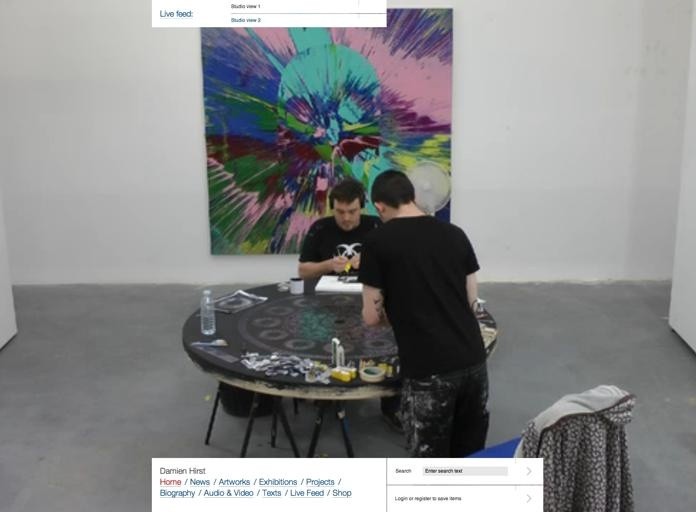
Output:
[460,383,639,511]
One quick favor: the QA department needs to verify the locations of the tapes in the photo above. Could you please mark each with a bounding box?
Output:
[358,366,386,383]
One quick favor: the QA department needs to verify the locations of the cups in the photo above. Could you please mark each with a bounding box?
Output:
[289,277,304,295]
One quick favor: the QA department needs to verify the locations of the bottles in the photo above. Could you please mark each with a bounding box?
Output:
[200,289,216,336]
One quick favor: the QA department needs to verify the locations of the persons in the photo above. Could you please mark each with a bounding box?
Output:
[297,178,409,434]
[356,166,490,458]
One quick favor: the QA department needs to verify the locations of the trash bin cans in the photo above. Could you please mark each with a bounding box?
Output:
[220,381,282,418]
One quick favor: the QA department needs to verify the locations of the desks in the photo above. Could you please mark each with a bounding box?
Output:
[182,275,498,458]
